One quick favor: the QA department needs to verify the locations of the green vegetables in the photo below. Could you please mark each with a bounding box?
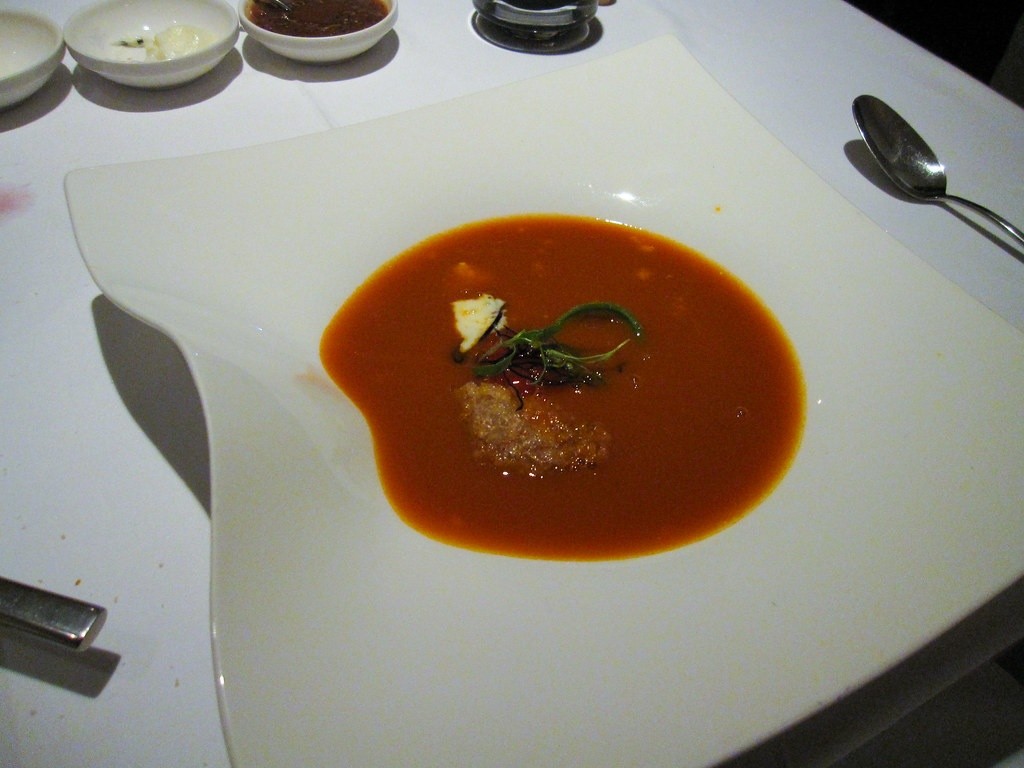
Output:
[469,303,644,386]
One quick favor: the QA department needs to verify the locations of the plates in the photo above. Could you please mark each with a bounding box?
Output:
[63,35,1024,768]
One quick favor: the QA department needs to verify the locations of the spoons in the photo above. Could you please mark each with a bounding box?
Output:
[852,94,1024,260]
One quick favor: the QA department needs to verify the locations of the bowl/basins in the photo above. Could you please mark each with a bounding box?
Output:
[238,0,399,62]
[0,6,66,109]
[62,0,240,89]
[469,0,598,54]
